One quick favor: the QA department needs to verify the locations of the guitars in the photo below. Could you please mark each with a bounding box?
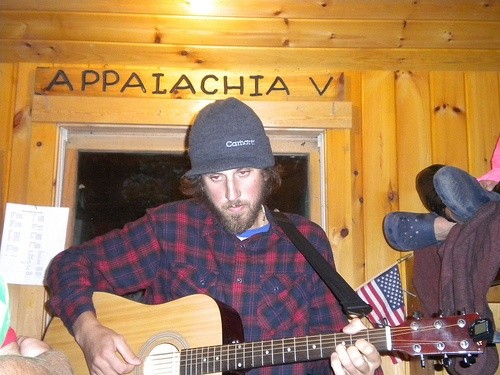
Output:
[39,288,491,375]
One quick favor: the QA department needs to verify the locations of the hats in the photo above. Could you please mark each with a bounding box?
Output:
[183,98,275,178]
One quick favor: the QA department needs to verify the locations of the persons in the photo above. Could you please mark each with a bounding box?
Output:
[0,327,72,375]
[383,134,500,252]
[44,96,385,375]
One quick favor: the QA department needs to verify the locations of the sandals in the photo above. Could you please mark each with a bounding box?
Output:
[433,167,490,223]
[383,212,445,252]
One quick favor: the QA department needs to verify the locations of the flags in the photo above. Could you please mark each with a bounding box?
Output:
[353,264,411,364]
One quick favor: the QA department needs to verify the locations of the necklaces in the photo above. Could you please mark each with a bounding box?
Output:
[259,213,266,227]
